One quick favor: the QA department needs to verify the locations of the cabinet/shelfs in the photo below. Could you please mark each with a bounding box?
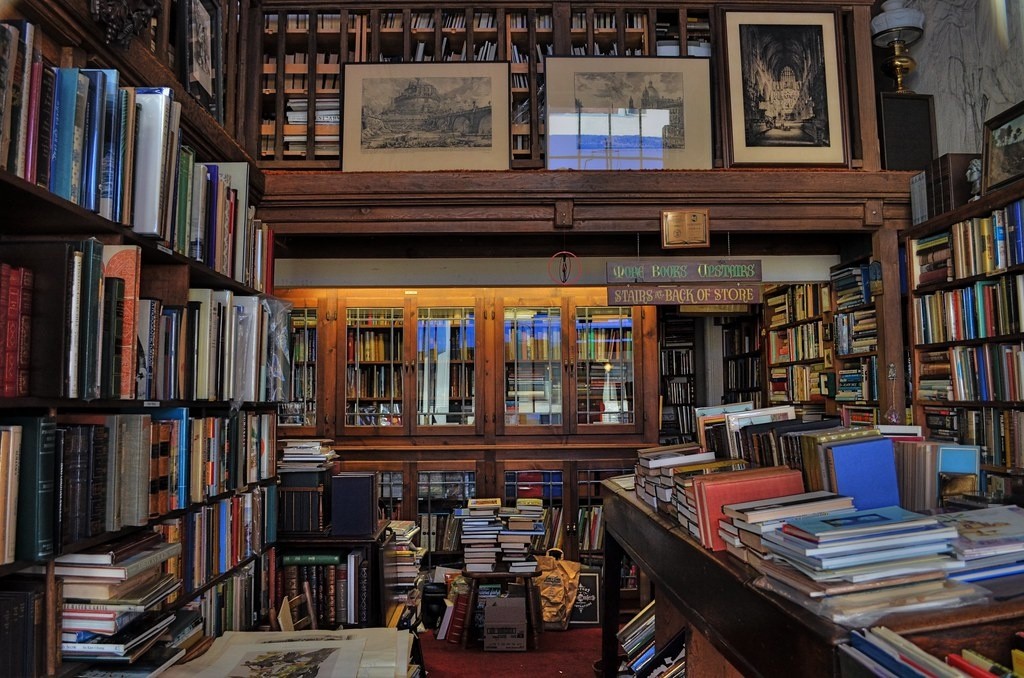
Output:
[722,314,763,409]
[255,0,884,173]
[658,343,695,441]
[764,255,879,427]
[275,298,659,618]
[280,519,394,631]
[902,178,1024,510]
[0,0,282,677]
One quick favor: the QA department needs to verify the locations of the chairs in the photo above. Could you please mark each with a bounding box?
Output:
[287,581,317,632]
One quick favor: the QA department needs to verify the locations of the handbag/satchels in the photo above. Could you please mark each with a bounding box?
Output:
[533,548,581,630]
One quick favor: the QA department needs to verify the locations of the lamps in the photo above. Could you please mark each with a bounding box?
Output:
[870,0,925,93]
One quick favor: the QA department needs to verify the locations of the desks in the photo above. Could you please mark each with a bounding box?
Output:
[594,482,1024,678]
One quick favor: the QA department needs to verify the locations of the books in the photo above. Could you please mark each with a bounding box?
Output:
[0,0,1024,678]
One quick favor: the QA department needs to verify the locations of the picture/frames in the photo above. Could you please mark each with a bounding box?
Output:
[712,1,853,170]
[982,98,1023,197]
[544,56,714,170]
[183,0,223,128]
[340,60,513,171]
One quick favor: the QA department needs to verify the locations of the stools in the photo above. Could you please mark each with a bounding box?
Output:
[460,566,542,652]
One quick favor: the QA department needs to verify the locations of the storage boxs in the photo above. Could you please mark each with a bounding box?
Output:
[484,596,527,651]
